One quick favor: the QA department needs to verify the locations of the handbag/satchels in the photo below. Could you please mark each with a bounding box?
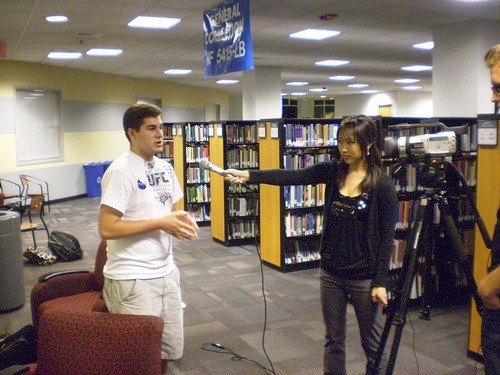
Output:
[23,245,57,265]
[48,232,82,261]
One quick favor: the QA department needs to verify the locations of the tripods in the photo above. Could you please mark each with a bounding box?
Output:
[369,158,491,375]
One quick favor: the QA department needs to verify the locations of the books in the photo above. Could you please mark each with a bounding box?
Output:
[184,123,211,222]
[284,123,340,264]
[225,123,261,240]
[155,124,175,169]
[374,121,477,300]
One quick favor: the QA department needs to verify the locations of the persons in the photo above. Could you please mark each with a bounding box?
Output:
[476,45,500,375]
[225,115,397,375]
[99,103,199,375]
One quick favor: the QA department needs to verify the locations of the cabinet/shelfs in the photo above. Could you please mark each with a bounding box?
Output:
[209,120,259,248]
[344,115,477,315]
[466,115,500,366]
[257,118,339,273]
[172,121,211,228]
[155,123,174,167]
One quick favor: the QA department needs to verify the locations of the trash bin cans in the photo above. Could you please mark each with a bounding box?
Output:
[83,160,113,198]
[0,210,26,314]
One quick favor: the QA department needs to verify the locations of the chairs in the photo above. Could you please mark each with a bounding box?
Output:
[0,174,167,375]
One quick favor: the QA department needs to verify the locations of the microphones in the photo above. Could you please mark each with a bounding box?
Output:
[199,158,253,192]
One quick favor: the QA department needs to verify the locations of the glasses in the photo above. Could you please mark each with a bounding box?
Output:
[490,84,500,95]
